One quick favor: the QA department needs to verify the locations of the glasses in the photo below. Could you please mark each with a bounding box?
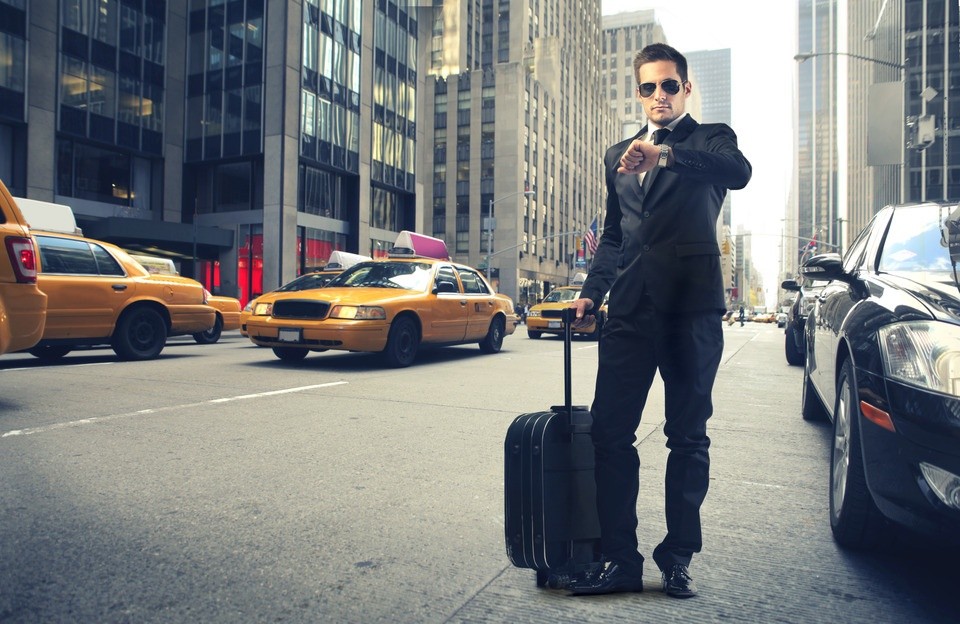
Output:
[637,80,687,98]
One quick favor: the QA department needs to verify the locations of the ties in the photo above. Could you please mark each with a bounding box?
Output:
[642,127,670,196]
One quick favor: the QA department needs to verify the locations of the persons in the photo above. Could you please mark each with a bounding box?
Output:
[515,301,532,324]
[577,44,752,595]
[738,305,744,327]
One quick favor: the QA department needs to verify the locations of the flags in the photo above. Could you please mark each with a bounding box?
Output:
[584,217,598,253]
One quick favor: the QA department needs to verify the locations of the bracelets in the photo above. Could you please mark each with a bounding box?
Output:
[658,144,668,168]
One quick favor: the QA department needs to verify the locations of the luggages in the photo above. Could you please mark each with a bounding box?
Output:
[503,307,607,588]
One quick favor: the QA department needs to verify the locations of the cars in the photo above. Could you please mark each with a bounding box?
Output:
[781,264,834,366]
[0,177,48,357]
[245,230,523,368]
[524,271,608,341]
[12,195,217,363]
[129,254,241,344]
[797,199,960,560]
[721,309,788,329]
[237,250,374,347]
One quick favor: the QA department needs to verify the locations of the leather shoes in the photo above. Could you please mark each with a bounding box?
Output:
[565,559,643,594]
[661,563,696,598]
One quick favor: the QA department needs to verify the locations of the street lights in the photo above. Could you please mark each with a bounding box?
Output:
[486,191,536,284]
[781,218,821,255]
[793,52,913,205]
